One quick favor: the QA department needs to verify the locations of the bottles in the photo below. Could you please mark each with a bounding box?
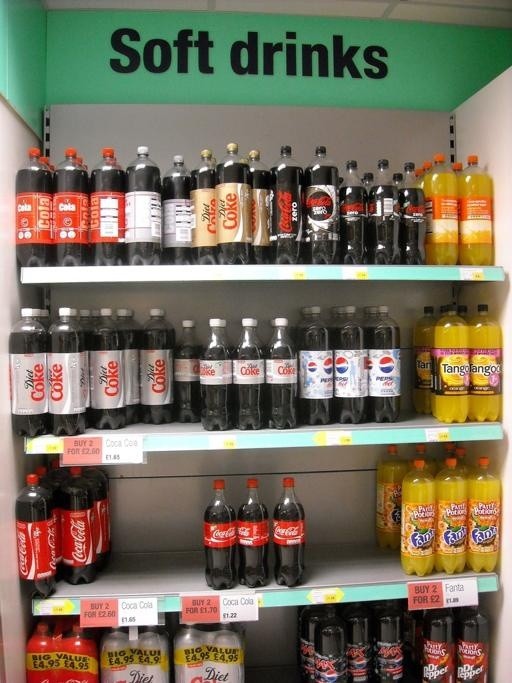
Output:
[373,444,503,578]
[17,145,501,268]
[15,460,113,599]
[201,476,308,592]
[26,594,491,683]
[13,303,504,428]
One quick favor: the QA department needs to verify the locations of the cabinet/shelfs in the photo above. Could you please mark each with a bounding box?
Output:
[19,261,507,617]
